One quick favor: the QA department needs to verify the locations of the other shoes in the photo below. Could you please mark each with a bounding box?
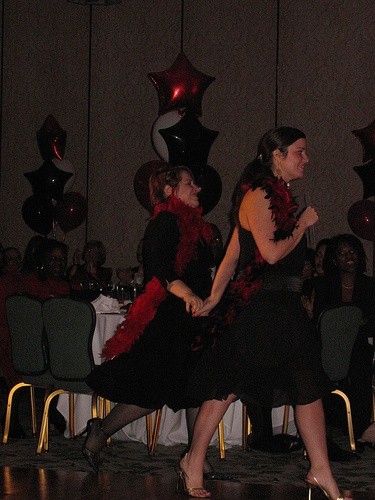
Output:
[1,414,27,439]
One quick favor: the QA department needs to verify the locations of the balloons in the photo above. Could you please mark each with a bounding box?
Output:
[133,52,223,218]
[348,121,375,245]
[23,114,89,235]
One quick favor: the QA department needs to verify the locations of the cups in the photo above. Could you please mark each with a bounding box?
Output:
[79,279,129,303]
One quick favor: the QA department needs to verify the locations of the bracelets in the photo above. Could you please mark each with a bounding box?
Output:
[129,279,135,285]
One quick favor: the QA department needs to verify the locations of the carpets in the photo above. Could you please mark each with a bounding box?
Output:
[0,401,375,493]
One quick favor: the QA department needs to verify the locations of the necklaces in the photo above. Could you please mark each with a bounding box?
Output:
[341,283,355,290]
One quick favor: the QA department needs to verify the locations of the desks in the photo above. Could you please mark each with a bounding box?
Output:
[55,307,298,451]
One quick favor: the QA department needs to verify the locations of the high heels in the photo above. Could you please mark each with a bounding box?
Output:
[174,452,213,498]
[183,447,233,480]
[303,469,353,500]
[79,416,112,474]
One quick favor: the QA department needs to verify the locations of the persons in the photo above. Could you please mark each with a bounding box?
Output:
[313,238,332,275]
[175,125,356,500]
[300,247,317,281]
[240,402,361,463]
[79,165,236,482]
[298,233,375,454]
[2,246,23,274]
[114,238,146,298]
[0,245,67,439]
[67,239,114,304]
[203,221,235,285]
[38,240,80,302]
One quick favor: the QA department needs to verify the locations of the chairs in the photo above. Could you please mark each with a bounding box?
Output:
[3,265,375,461]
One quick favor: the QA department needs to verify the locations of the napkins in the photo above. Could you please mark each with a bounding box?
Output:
[92,294,121,314]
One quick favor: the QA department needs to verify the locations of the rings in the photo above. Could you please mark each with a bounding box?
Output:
[186,302,190,305]
